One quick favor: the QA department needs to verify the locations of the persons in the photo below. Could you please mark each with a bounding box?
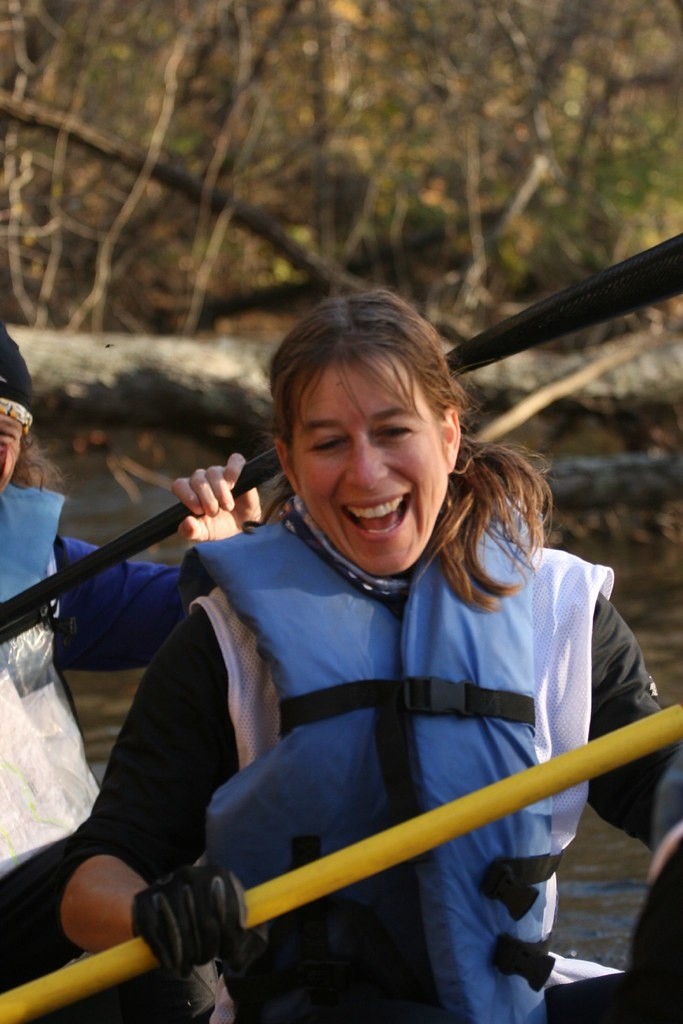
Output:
[0,322,262,1024]
[53,289,683,1024]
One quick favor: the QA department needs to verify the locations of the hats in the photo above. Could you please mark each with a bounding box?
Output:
[1,323,34,433]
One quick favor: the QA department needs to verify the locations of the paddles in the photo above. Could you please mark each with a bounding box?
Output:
[0,237,683,644]
[0,701,683,1024]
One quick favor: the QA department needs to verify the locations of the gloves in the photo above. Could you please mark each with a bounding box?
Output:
[132,866,265,978]
